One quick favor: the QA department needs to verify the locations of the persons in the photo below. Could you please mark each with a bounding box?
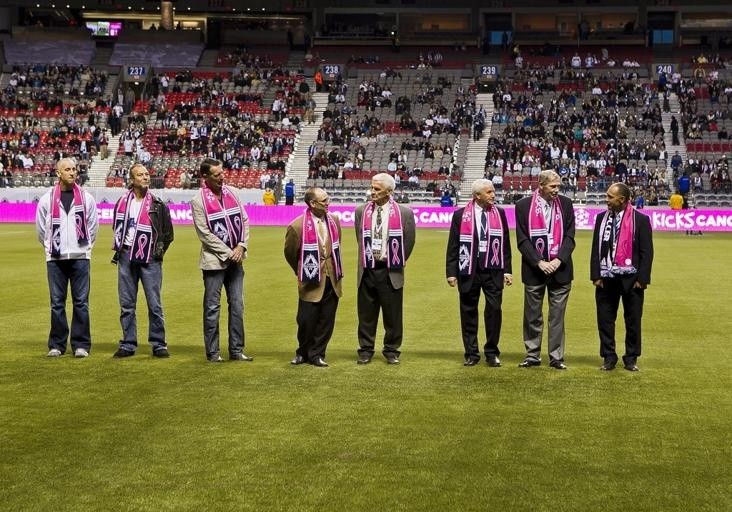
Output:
[110,163,173,358]
[190,158,253,362]
[36,158,100,357]
[516,170,575,369]
[355,173,416,364]
[284,186,343,367]
[446,179,513,367]
[591,182,653,371]
[2,11,729,207]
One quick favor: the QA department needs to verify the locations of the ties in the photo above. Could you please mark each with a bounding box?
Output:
[544,203,552,233]
[479,209,488,271]
[615,213,622,244]
[318,220,324,242]
[373,207,383,261]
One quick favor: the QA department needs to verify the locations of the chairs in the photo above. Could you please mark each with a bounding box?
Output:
[104,69,306,188]
[486,67,671,204]
[309,66,477,203]
[0,63,123,186]
[679,82,732,207]
